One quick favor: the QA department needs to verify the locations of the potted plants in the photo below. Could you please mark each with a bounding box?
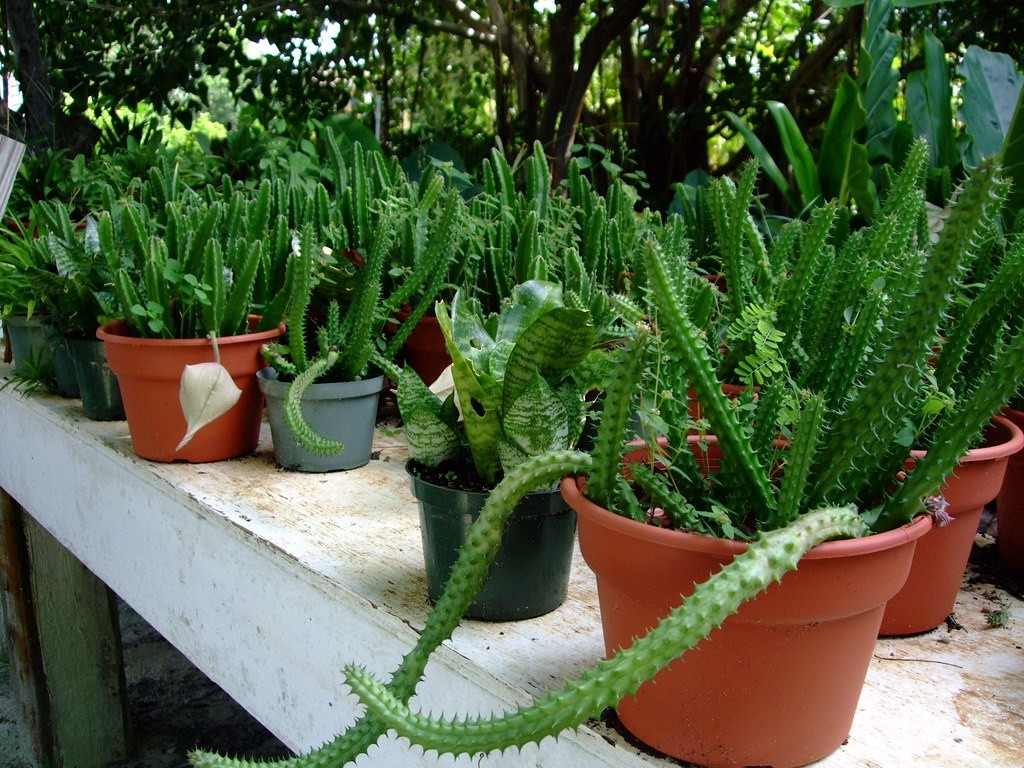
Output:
[50,217,125,422]
[681,145,845,435]
[395,171,475,425]
[0,205,48,379]
[96,188,287,463]
[398,278,616,622]
[184,134,1024,768]
[43,218,80,400]
[674,175,733,326]
[830,149,1024,637]
[255,184,461,473]
